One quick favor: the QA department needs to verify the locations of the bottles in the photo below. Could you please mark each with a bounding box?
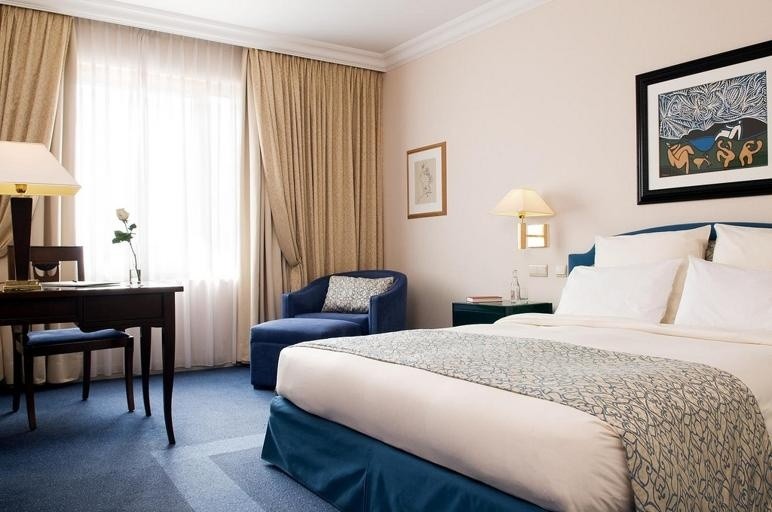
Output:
[510,269,520,302]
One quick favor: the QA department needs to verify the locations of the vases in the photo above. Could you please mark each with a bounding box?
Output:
[129,243,143,287]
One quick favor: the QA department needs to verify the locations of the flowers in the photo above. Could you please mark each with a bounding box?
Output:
[111,209,141,282]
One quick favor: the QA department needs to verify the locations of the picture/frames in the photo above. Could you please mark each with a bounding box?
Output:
[406,141,448,220]
[634,39,771,205]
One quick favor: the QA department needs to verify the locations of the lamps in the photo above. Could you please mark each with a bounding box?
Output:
[1,140,82,282]
[493,188,555,250]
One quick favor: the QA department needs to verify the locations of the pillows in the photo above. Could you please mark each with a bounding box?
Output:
[554,258,686,325]
[321,275,395,313]
[592,224,712,325]
[672,253,770,343]
[712,222,771,275]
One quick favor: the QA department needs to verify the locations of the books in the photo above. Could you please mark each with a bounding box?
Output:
[3,280,41,293]
[466,296,503,302]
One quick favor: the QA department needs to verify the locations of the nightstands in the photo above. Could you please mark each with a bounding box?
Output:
[451,300,553,328]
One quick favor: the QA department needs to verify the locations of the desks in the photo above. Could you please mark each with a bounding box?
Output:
[0,283,186,446]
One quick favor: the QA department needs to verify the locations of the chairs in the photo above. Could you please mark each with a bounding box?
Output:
[7,243,136,431]
[249,268,408,389]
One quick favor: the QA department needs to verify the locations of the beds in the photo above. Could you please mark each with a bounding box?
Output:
[261,220,770,511]
